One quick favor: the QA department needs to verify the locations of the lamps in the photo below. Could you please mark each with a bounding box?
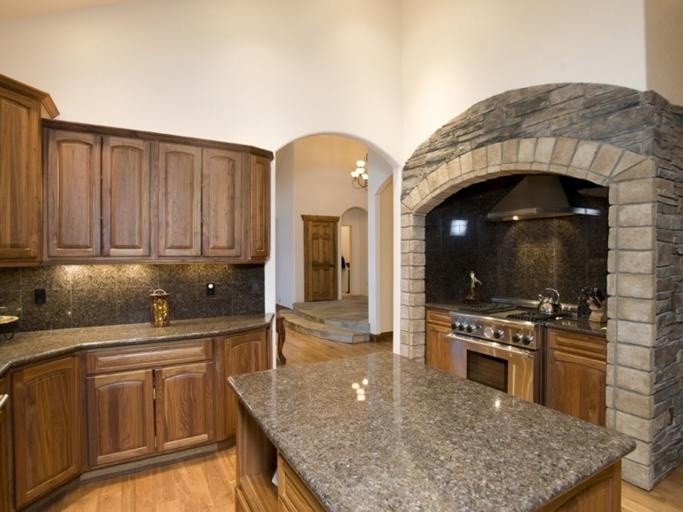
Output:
[349,153,368,192]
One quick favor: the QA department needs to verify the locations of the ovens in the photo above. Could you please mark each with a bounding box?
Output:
[450,333,542,403]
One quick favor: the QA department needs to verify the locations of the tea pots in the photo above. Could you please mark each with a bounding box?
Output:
[537,287,562,314]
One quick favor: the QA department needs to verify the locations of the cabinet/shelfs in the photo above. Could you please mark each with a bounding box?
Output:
[218,328,268,449]
[236,397,326,511]
[425,307,451,374]
[250,146,273,265]
[150,132,251,265]
[0,74,45,268]
[0,352,82,512]
[42,118,150,263]
[542,326,607,427]
[82,335,218,481]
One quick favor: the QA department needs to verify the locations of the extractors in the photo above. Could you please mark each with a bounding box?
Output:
[483,175,578,222]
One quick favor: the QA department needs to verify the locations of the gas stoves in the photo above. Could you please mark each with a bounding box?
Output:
[450,301,567,349]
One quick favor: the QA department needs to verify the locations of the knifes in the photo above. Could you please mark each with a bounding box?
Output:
[585,287,604,305]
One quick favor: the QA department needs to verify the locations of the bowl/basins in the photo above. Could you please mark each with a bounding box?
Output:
[0,314,19,333]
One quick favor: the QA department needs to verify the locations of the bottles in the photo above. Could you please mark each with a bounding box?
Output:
[577,286,591,318]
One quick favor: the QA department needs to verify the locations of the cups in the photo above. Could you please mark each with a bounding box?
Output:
[148,290,171,329]
